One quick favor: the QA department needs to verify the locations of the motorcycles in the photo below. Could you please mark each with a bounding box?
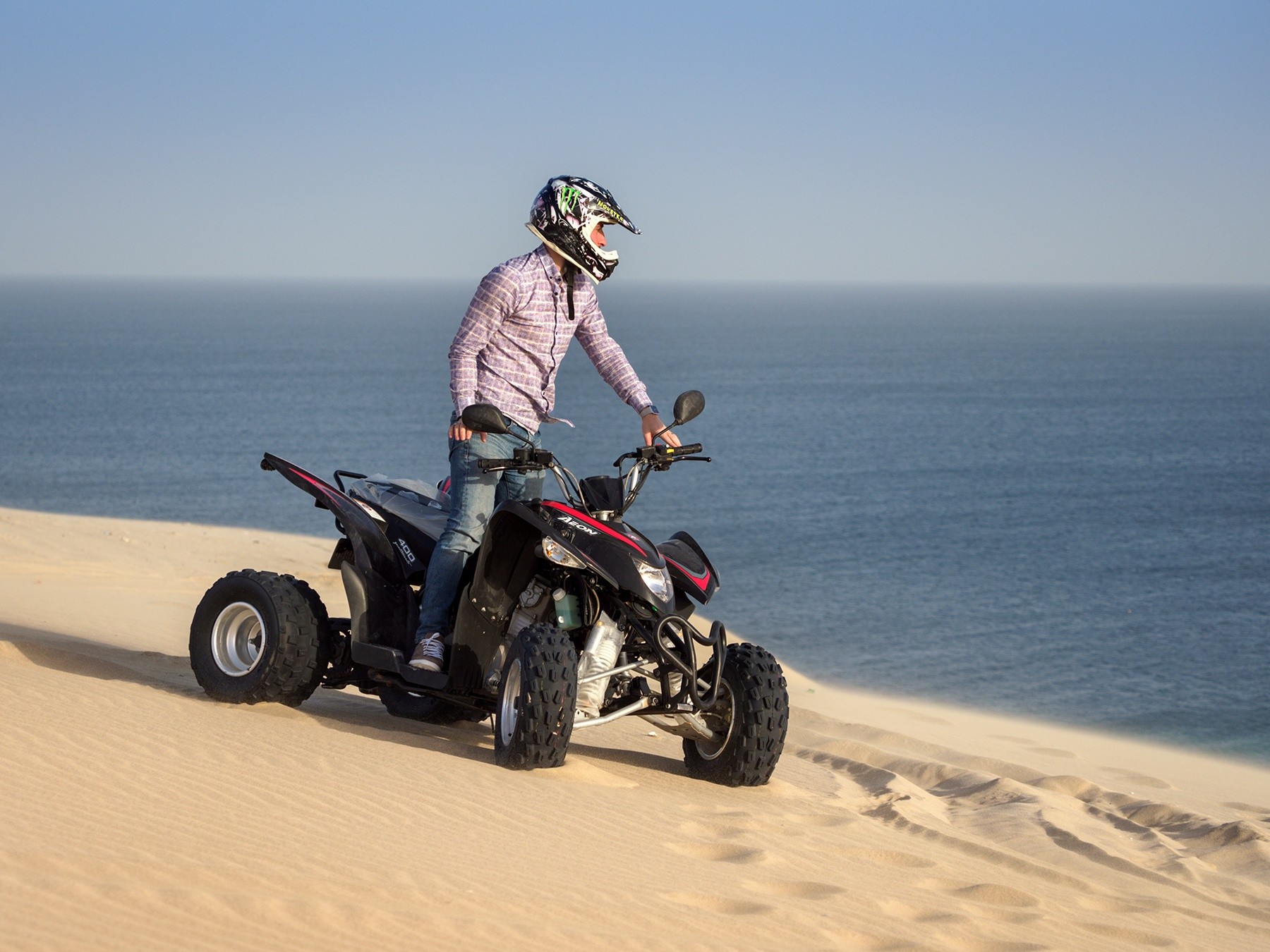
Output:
[186,388,788,786]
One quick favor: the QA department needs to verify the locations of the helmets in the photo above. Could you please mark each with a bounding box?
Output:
[525,175,643,285]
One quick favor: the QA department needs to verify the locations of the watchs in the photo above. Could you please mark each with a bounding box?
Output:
[640,405,659,418]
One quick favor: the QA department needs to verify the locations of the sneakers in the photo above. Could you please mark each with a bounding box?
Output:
[409,633,444,672]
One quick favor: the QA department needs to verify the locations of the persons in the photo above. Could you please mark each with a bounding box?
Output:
[409,176,681,676]
